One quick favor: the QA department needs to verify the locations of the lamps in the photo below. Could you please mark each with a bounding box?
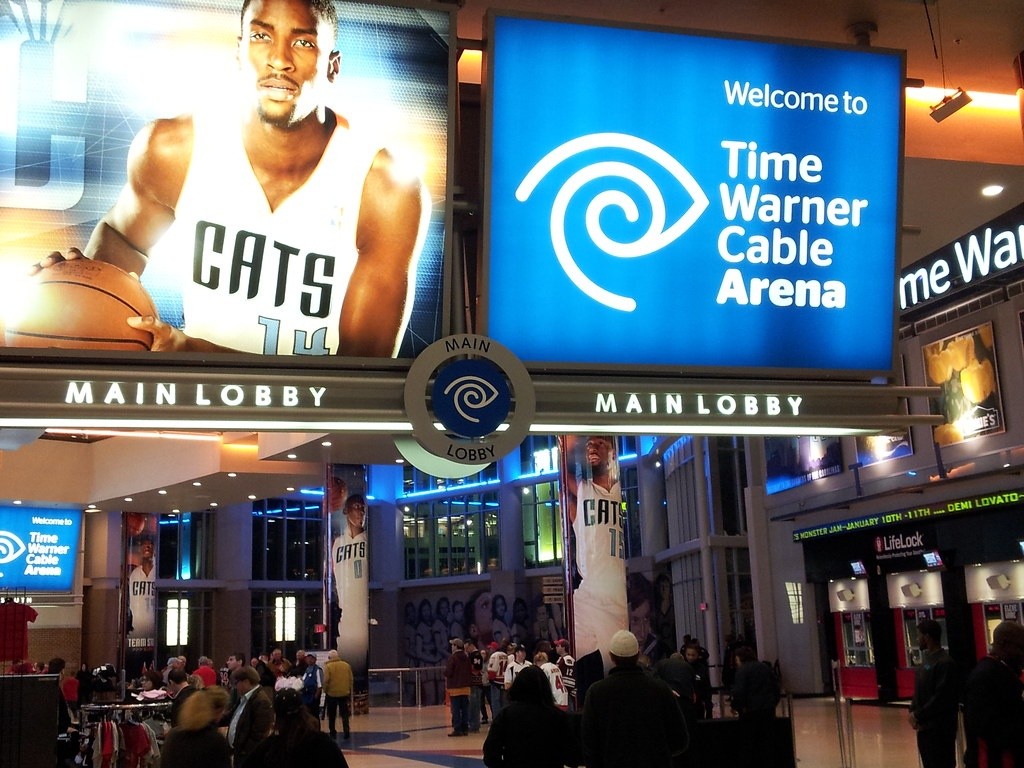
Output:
[928,87,971,124]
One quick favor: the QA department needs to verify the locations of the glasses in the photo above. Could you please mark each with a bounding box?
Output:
[140,678,149,682]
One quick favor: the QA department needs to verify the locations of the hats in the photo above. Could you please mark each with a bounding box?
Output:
[304,652,316,659]
[273,686,303,714]
[553,639,570,648]
[487,642,501,649]
[449,638,463,647]
[609,629,638,657]
[515,644,526,651]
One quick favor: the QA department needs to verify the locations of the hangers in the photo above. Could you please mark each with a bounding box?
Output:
[94,705,167,722]
[1,587,13,605]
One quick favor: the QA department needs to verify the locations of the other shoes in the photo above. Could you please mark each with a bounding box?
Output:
[464,732,467,736]
[448,731,463,736]
[468,727,479,732]
[481,719,488,724]
[343,732,350,739]
[330,730,337,739]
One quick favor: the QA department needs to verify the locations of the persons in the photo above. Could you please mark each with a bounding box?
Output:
[444,629,781,768]
[907,618,1024,768]
[27,0,421,360]
[129,539,157,637]
[0,649,353,768]
[332,434,673,682]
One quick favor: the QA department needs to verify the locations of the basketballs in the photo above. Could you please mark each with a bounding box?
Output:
[126,512,147,536]
[328,476,349,512]
[4,258,160,352]
